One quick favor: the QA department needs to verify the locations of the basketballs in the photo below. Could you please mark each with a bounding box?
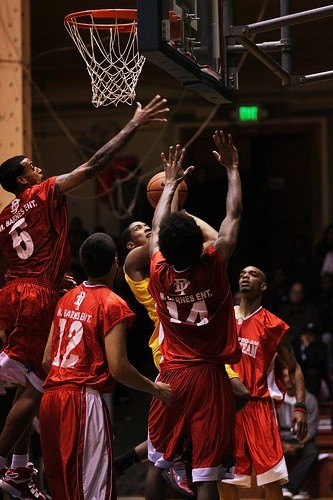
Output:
[146,171,188,209]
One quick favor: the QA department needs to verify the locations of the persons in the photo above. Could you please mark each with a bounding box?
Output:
[39,231,173,500]
[114,178,250,496]
[0,94,170,500]
[62,213,333,500]
[145,129,243,500]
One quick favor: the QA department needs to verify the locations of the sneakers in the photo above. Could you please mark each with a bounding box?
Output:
[0,463,46,500]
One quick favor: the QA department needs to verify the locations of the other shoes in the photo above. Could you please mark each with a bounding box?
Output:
[161,455,195,497]
[282,484,309,500]
